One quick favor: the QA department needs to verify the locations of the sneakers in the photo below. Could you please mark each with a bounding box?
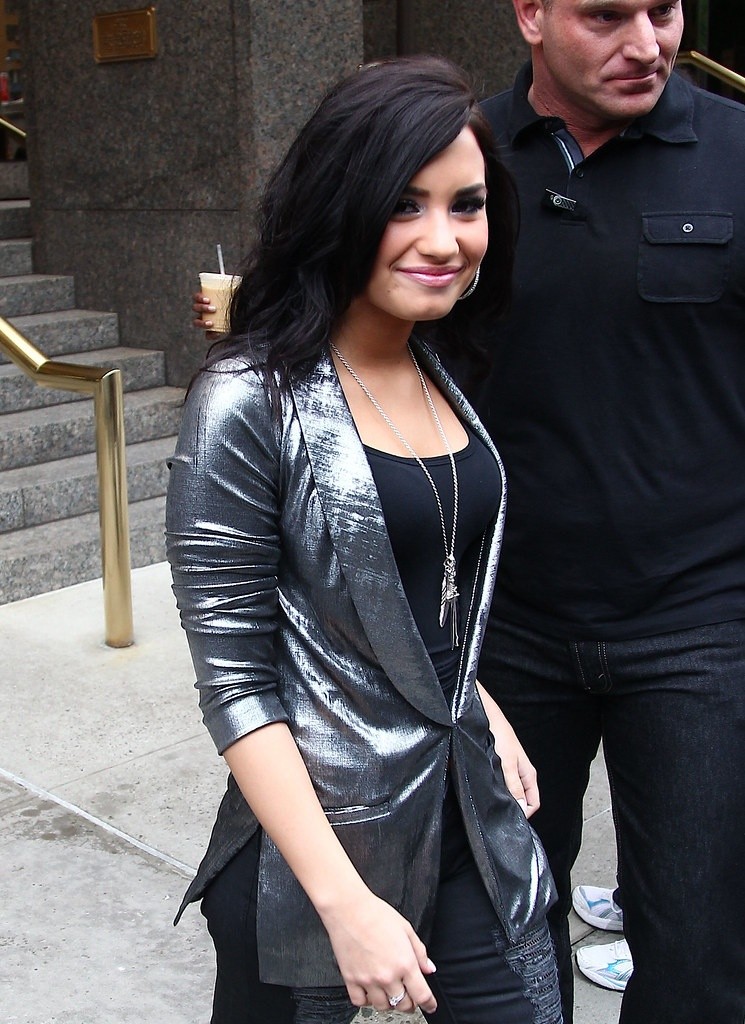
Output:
[575,939,633,991]
[572,885,623,931]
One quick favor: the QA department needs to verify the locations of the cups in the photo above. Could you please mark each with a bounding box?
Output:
[198,272,243,331]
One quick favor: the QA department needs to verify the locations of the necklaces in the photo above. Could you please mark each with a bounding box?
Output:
[331,339,462,653]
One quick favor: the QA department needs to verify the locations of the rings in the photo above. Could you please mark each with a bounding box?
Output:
[389,990,405,1007]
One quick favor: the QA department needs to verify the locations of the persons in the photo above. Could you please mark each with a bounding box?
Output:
[166,59,569,1024]
[191,0,745,1024]
